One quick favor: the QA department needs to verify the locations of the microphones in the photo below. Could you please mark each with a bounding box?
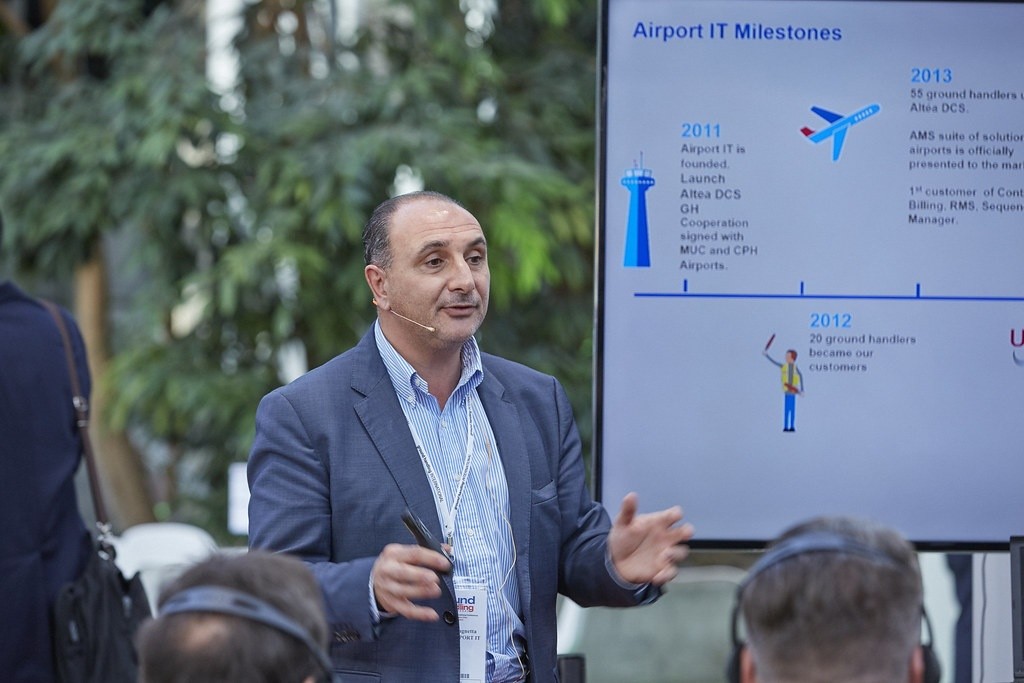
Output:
[390,313,435,332]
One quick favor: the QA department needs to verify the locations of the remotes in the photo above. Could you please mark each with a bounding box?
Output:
[401,509,454,574]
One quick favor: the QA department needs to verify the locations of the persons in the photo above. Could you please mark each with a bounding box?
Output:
[1,280,108,682]
[248,189,696,683]
[136,551,333,682]
[737,518,925,683]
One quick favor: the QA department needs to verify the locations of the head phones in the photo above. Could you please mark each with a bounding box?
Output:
[151,584,341,683]
[724,532,948,683]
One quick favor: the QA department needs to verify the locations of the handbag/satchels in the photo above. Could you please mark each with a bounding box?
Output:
[32,299,154,683]
